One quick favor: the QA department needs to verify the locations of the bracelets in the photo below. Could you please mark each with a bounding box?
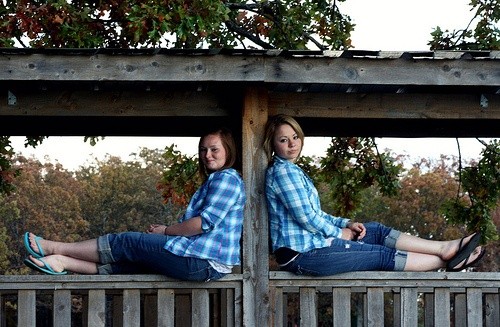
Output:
[164,226,168,235]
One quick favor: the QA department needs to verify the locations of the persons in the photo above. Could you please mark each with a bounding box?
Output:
[263,114,485,279]
[24,126,245,282]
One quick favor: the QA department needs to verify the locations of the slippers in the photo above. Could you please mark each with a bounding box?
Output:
[447,233,486,273]
[24,232,67,275]
